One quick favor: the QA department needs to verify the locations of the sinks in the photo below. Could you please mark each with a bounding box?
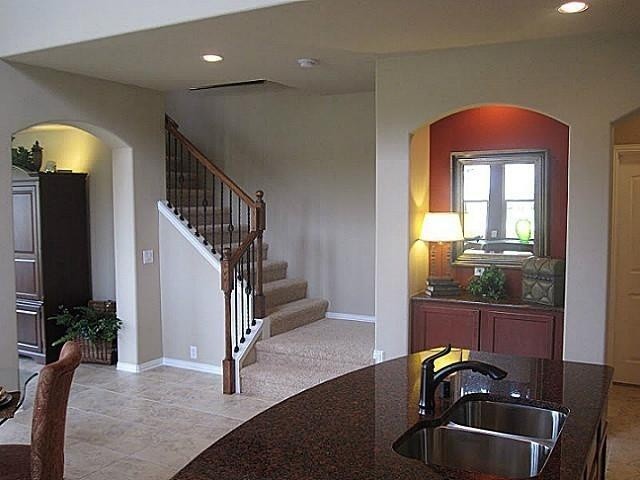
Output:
[388,420,553,480]
[440,390,575,443]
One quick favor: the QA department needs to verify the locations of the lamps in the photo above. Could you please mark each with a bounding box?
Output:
[419,212,465,297]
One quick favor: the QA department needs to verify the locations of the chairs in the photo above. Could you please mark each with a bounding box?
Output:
[0,340,82,479]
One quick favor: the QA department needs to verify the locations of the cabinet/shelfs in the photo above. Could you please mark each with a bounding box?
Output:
[412,301,563,360]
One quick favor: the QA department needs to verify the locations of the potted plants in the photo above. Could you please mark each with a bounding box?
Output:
[47,306,122,364]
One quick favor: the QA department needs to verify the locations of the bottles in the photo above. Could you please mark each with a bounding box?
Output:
[516,219,532,243]
[30,140,43,172]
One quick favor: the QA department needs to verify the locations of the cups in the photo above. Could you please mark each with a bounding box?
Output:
[45,160,56,174]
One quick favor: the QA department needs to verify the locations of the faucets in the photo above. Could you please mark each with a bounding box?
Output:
[416,341,510,419]
